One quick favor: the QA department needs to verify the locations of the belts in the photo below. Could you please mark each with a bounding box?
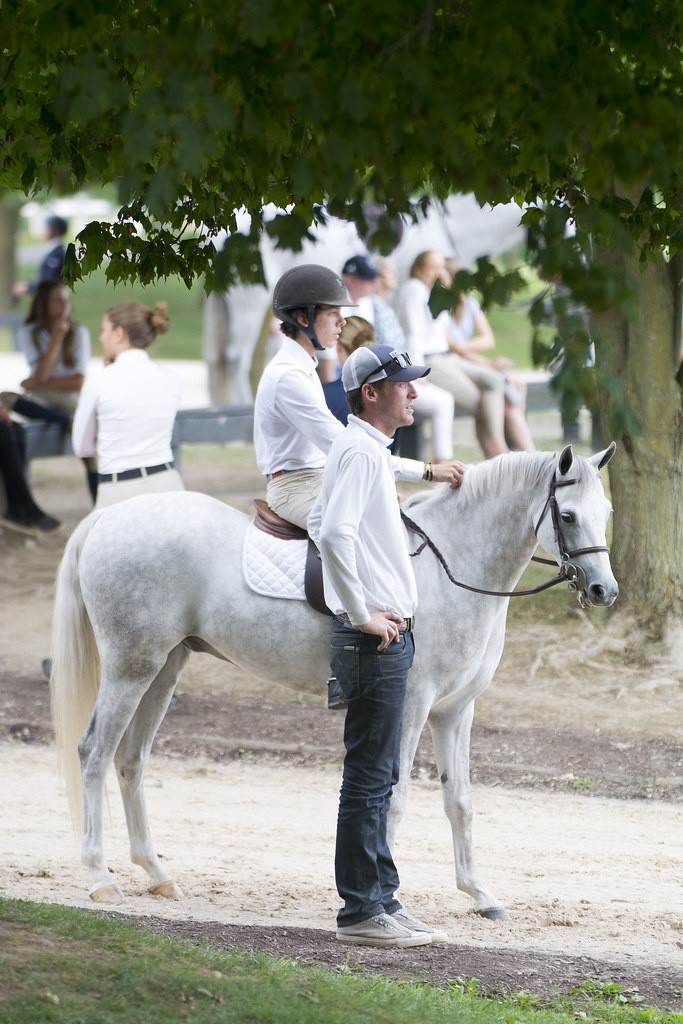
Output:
[266,468,291,483]
[96,461,172,481]
[338,612,416,632]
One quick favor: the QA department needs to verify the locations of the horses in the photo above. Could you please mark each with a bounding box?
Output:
[47,440,626,926]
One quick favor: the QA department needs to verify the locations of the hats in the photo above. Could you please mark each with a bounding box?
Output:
[343,255,382,281]
[342,344,432,393]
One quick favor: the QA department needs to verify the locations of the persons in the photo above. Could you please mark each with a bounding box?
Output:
[251,264,464,708]
[201,251,601,470]
[12,216,69,325]
[17,278,99,506]
[0,399,64,539]
[305,347,447,950]
[43,301,185,682]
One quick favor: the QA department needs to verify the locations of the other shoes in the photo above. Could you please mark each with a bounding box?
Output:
[326,674,351,711]
[1,506,65,537]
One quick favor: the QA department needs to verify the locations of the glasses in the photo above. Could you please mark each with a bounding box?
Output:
[360,352,412,391]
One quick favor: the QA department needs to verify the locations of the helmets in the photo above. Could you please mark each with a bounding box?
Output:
[272,263,359,321]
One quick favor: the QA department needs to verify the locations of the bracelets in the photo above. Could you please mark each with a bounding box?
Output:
[426,462,433,482]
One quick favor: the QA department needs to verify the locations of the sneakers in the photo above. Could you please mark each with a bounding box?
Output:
[336,913,432,948]
[388,909,448,943]
